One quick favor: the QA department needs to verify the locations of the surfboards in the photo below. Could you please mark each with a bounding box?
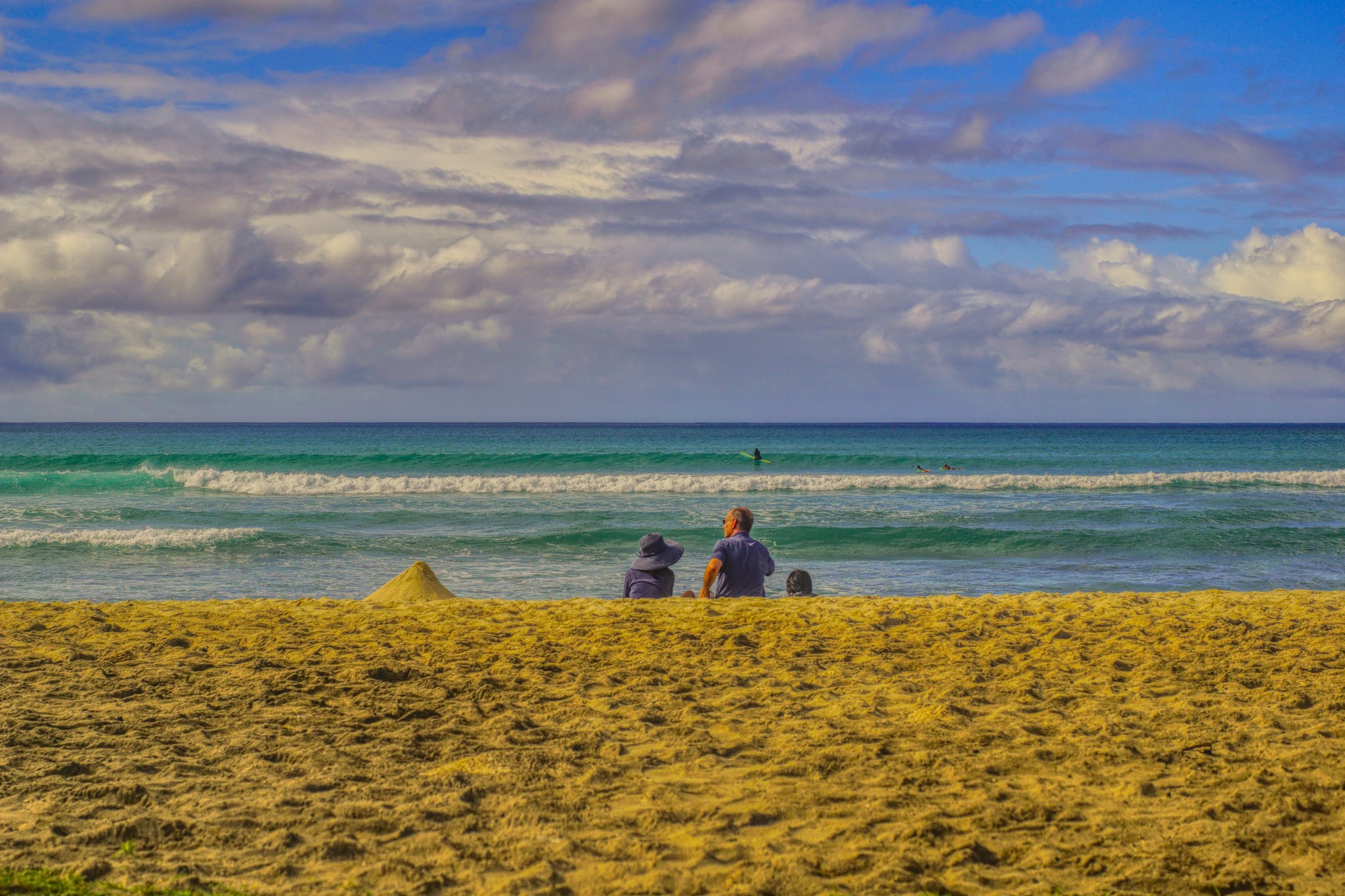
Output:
[940,467,956,470]
[740,452,771,465]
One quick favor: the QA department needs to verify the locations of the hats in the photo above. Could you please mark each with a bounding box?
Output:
[629,533,684,569]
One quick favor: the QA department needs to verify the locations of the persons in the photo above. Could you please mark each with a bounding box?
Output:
[697,508,775,598]
[752,448,762,460]
[944,464,962,471]
[786,569,819,597]
[914,466,930,473]
[623,533,695,600]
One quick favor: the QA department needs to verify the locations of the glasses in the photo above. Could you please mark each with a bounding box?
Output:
[723,519,733,524]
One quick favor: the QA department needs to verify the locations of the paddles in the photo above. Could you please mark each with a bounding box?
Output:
[912,465,917,469]
